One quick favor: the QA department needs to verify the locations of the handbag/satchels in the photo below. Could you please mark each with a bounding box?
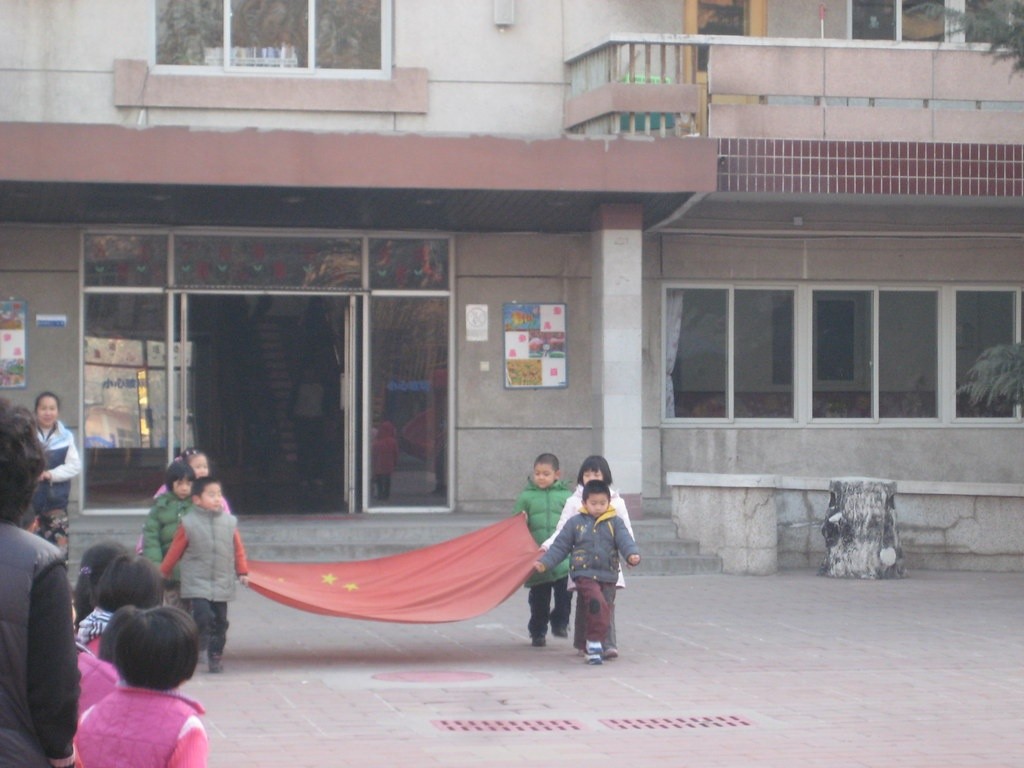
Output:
[40,510,68,544]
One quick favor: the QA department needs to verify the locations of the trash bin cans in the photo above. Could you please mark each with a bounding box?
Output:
[818,476,909,581]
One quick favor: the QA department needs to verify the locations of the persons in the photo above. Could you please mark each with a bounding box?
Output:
[28,392,84,567]
[142,460,197,612]
[538,455,635,657]
[75,605,209,768]
[71,607,142,723]
[514,453,574,646]
[533,480,641,666]
[0,397,81,768]
[372,421,399,498]
[160,477,251,673]
[76,554,162,658]
[73,542,131,633]
[136,447,231,554]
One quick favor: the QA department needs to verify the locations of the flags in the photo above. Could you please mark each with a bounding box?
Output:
[243,510,545,623]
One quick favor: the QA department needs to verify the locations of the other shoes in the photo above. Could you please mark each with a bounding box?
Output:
[585,655,600,664]
[603,646,618,659]
[551,614,568,638]
[587,640,602,653]
[208,651,222,672]
[532,634,546,645]
